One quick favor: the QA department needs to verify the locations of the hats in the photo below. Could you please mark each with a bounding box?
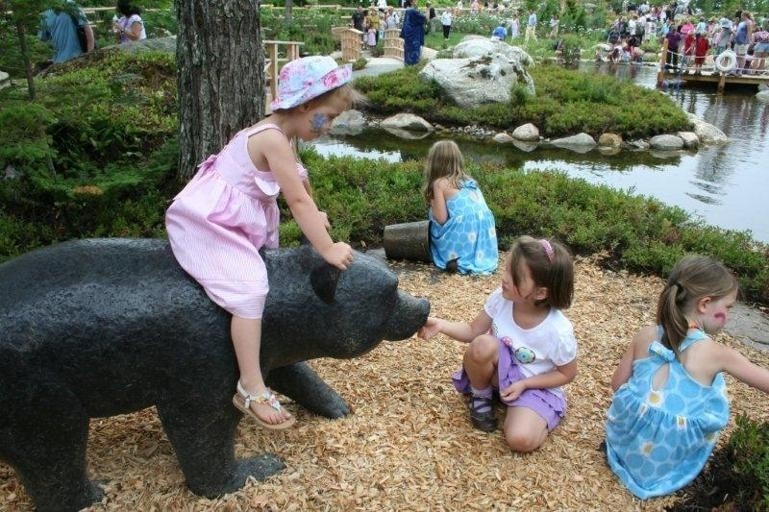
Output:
[270,56,354,111]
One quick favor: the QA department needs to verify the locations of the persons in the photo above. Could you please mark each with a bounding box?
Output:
[160,54,363,428]
[354,0,400,49]
[399,0,428,66]
[492,8,559,47]
[669,85,769,141]
[422,139,498,279]
[112,0,147,44]
[36,0,96,65]
[425,1,480,39]
[602,252,769,502]
[417,234,579,451]
[596,0,769,77]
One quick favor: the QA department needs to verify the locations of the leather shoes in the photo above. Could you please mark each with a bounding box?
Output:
[470,397,497,431]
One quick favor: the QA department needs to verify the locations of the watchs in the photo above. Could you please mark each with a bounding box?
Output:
[120,27,125,34]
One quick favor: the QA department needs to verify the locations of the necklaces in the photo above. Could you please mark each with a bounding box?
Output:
[686,323,700,331]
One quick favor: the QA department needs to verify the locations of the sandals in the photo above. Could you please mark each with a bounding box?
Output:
[233,382,295,430]
